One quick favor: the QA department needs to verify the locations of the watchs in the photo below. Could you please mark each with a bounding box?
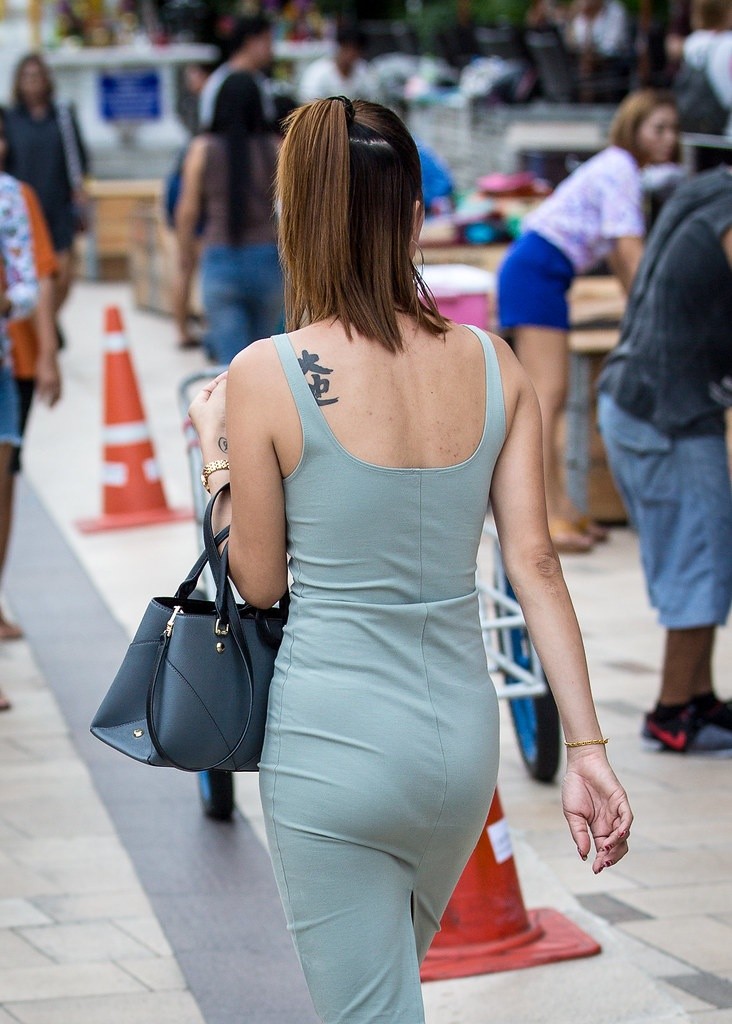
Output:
[200,459,229,493]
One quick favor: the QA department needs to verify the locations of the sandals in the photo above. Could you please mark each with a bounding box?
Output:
[549,507,609,551]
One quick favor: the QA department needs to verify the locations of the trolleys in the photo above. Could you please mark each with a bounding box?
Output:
[178,364,566,821]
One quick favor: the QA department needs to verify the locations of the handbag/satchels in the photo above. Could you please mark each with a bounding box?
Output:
[87,481,286,773]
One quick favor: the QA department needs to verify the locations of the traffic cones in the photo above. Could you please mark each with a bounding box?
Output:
[70,302,196,535]
[419,782,602,983]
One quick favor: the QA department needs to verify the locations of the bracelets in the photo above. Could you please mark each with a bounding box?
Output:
[564,738,610,746]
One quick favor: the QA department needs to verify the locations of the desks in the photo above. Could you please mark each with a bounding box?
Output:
[390,243,633,535]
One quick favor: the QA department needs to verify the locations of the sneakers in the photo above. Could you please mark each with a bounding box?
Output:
[636,693,732,757]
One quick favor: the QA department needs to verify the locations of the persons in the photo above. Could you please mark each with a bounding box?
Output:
[0,0,732,756]
[188,97,632,1024]
